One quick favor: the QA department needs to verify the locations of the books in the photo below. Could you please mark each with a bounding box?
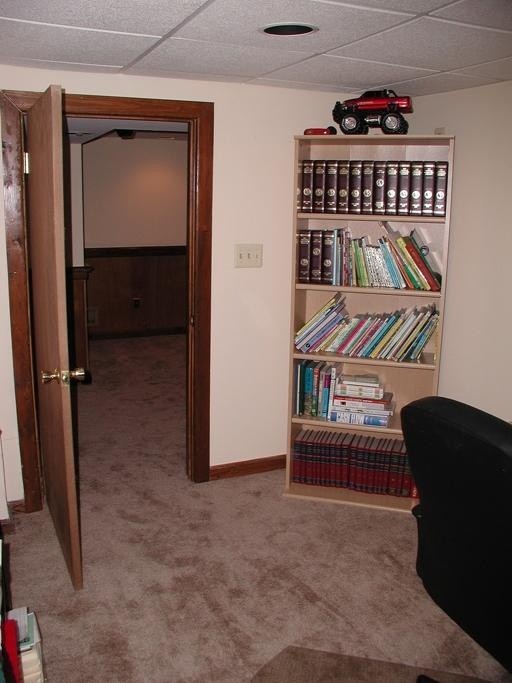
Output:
[294,292,439,362]
[293,428,417,498]
[7,606,49,683]
[297,359,395,427]
[297,160,447,216]
[297,221,443,291]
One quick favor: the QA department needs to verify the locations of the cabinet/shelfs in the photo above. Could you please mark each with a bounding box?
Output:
[281,133,455,515]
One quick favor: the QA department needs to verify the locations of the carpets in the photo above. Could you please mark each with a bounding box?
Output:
[250,645,486,683]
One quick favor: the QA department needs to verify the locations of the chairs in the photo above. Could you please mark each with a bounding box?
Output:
[400,395,511,672]
